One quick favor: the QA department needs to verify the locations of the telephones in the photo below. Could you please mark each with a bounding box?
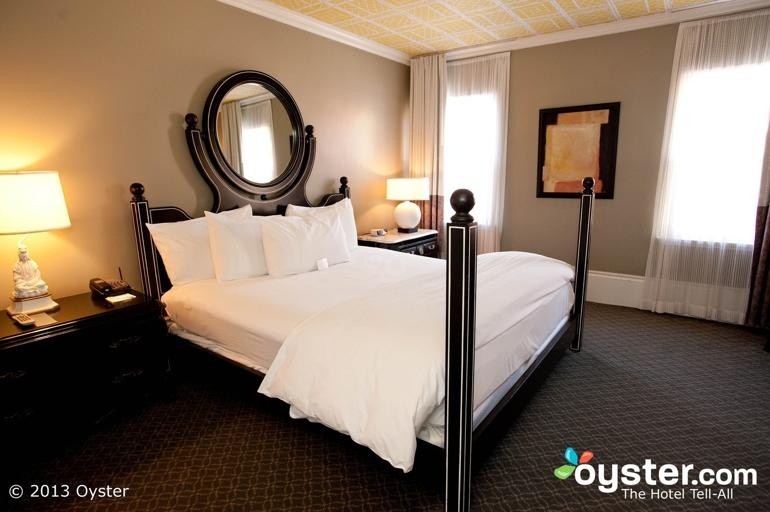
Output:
[89,267,131,298]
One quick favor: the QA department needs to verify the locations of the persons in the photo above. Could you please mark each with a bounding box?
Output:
[12,243,46,291]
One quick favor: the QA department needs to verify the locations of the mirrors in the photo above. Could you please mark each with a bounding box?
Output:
[184,70,316,216]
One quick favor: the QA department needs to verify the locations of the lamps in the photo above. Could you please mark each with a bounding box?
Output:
[1,171,72,235]
[386,178,430,233]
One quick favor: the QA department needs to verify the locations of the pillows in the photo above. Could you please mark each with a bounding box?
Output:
[145,197,359,286]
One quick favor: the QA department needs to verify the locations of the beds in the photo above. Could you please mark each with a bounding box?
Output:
[130,177,595,512]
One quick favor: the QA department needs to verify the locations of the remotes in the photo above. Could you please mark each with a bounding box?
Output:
[12,312,37,327]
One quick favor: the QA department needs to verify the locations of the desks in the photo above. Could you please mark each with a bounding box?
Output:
[358,227,439,259]
[0,288,167,440]
